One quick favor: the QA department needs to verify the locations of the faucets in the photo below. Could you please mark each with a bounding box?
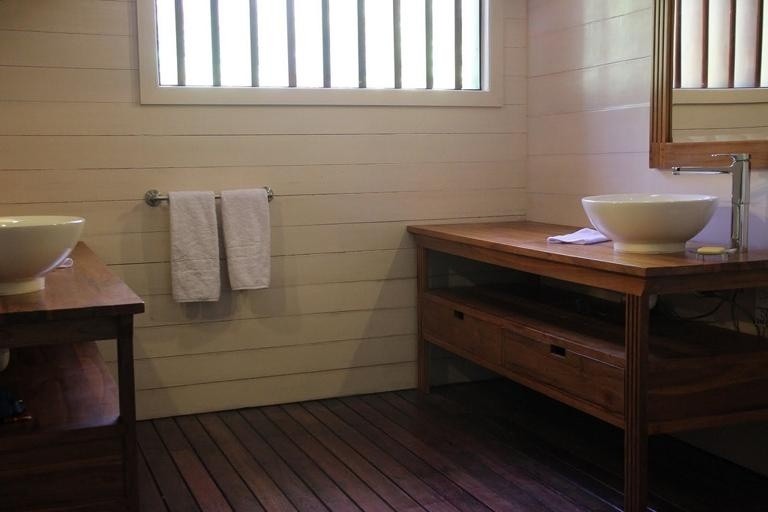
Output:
[671,152,751,253]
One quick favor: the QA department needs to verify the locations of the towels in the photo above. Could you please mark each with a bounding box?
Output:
[170,187,272,304]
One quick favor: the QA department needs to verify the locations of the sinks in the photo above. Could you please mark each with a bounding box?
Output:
[0,215,86,295]
[580,194,721,255]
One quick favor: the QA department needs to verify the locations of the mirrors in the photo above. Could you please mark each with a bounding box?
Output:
[648,0,768,170]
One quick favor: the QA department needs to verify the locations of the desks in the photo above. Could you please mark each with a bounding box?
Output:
[0,243,145,512]
[409,221,768,512]
